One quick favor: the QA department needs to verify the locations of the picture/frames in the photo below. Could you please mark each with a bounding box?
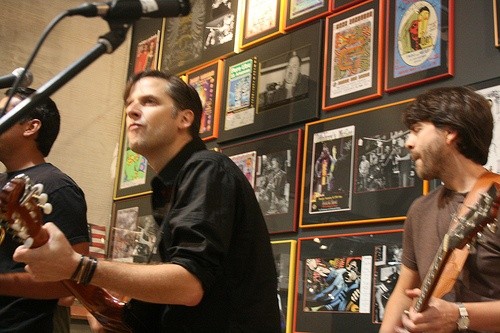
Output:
[216,18,324,145]
[269,240,297,333]
[321,0,383,111]
[238,0,286,51]
[218,127,304,234]
[292,229,407,333]
[299,98,429,228]
[284,0,333,32]
[111,106,155,202]
[384,0,455,93]
[185,59,224,141]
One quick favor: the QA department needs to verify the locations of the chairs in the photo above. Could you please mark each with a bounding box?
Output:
[65,223,108,322]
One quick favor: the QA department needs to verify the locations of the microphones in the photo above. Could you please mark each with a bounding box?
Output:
[0,68,34,89]
[68,0,191,19]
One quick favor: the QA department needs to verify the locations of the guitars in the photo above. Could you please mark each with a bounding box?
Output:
[0,173,132,333]
[397,182,500,312]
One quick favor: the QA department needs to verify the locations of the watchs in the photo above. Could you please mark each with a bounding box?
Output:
[455,301,471,333]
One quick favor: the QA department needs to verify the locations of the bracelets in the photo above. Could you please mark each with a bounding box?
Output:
[71,254,97,287]
[314,266,318,271]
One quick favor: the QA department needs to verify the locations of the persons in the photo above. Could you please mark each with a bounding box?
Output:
[13,69,284,333]
[0,85,91,333]
[305,132,419,323]
[379,85,500,333]
[266,52,312,103]
[255,151,288,214]
[134,40,155,74]
